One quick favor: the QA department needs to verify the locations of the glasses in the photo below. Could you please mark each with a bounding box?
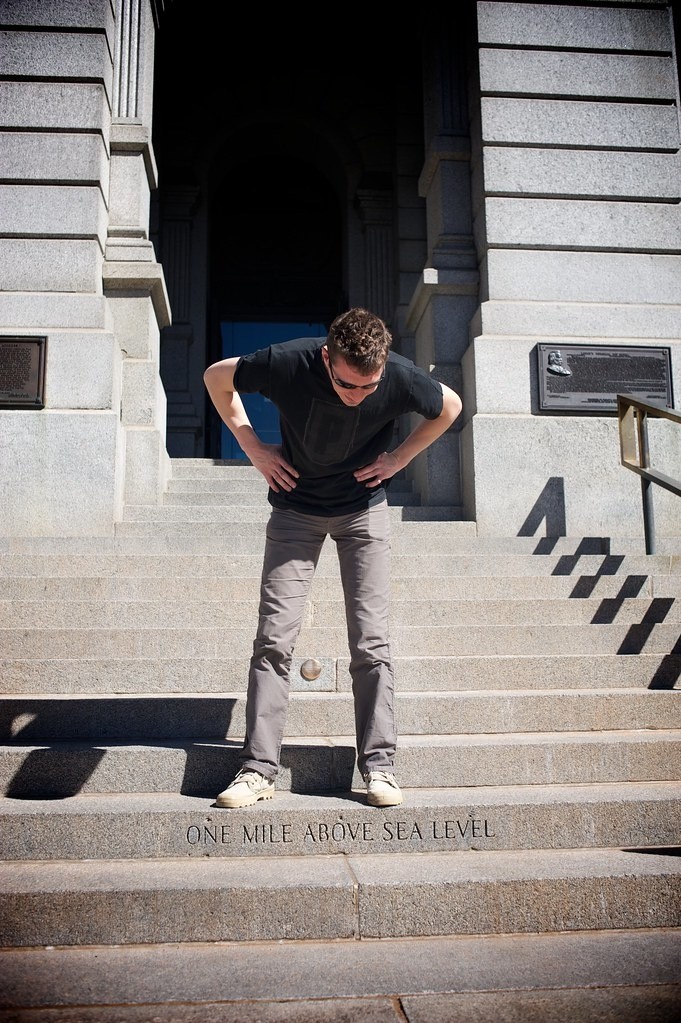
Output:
[327,344,386,390]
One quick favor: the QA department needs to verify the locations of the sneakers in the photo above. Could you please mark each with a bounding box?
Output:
[363,769,403,806]
[218,767,275,809]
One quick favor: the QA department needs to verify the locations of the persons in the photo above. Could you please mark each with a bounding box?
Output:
[203,307,462,808]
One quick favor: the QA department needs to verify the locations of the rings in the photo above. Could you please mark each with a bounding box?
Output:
[377,480,381,483]
